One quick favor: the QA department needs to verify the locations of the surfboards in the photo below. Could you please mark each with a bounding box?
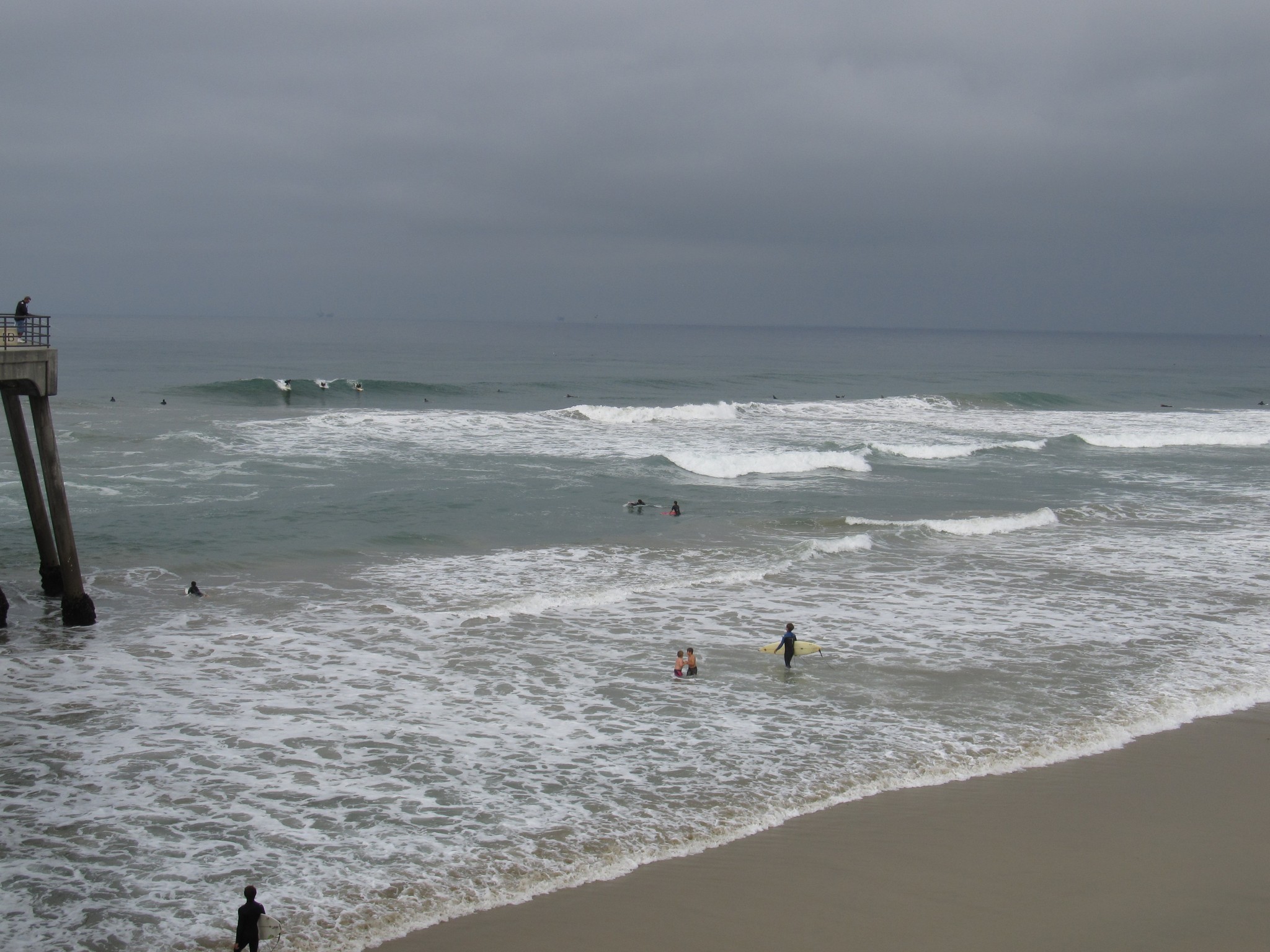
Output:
[758,640,821,656]
[258,913,283,940]
[185,588,191,594]
[661,512,675,514]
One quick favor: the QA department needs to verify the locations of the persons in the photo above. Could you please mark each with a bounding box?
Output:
[321,382,326,386]
[110,397,115,402]
[284,380,291,387]
[354,383,362,389]
[686,647,698,676]
[1160,404,1172,407]
[629,499,645,506]
[773,395,777,399]
[497,389,502,392]
[566,394,578,398]
[674,650,687,678]
[669,501,681,516]
[160,399,166,405]
[774,623,797,669]
[234,885,265,952]
[881,395,884,399]
[835,395,845,398]
[187,581,210,597]
[1258,401,1265,405]
[15,296,32,343]
[424,398,427,402]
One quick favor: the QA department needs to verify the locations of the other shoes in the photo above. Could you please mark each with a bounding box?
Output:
[17,338,25,343]
